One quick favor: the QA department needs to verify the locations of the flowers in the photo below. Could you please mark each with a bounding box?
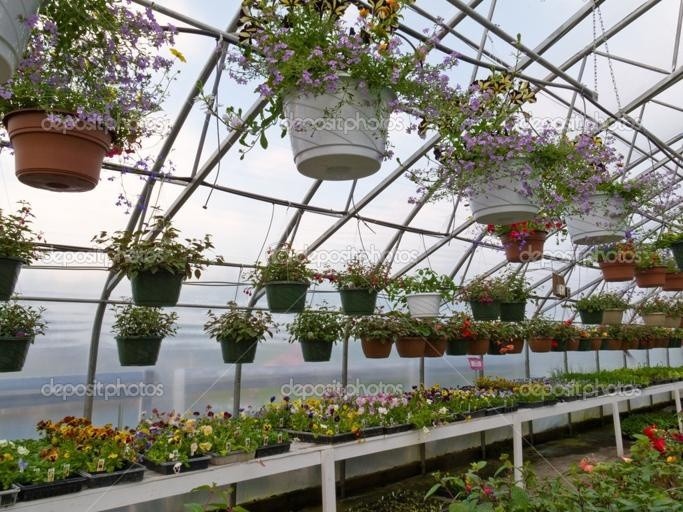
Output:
[0,2,682,510]
